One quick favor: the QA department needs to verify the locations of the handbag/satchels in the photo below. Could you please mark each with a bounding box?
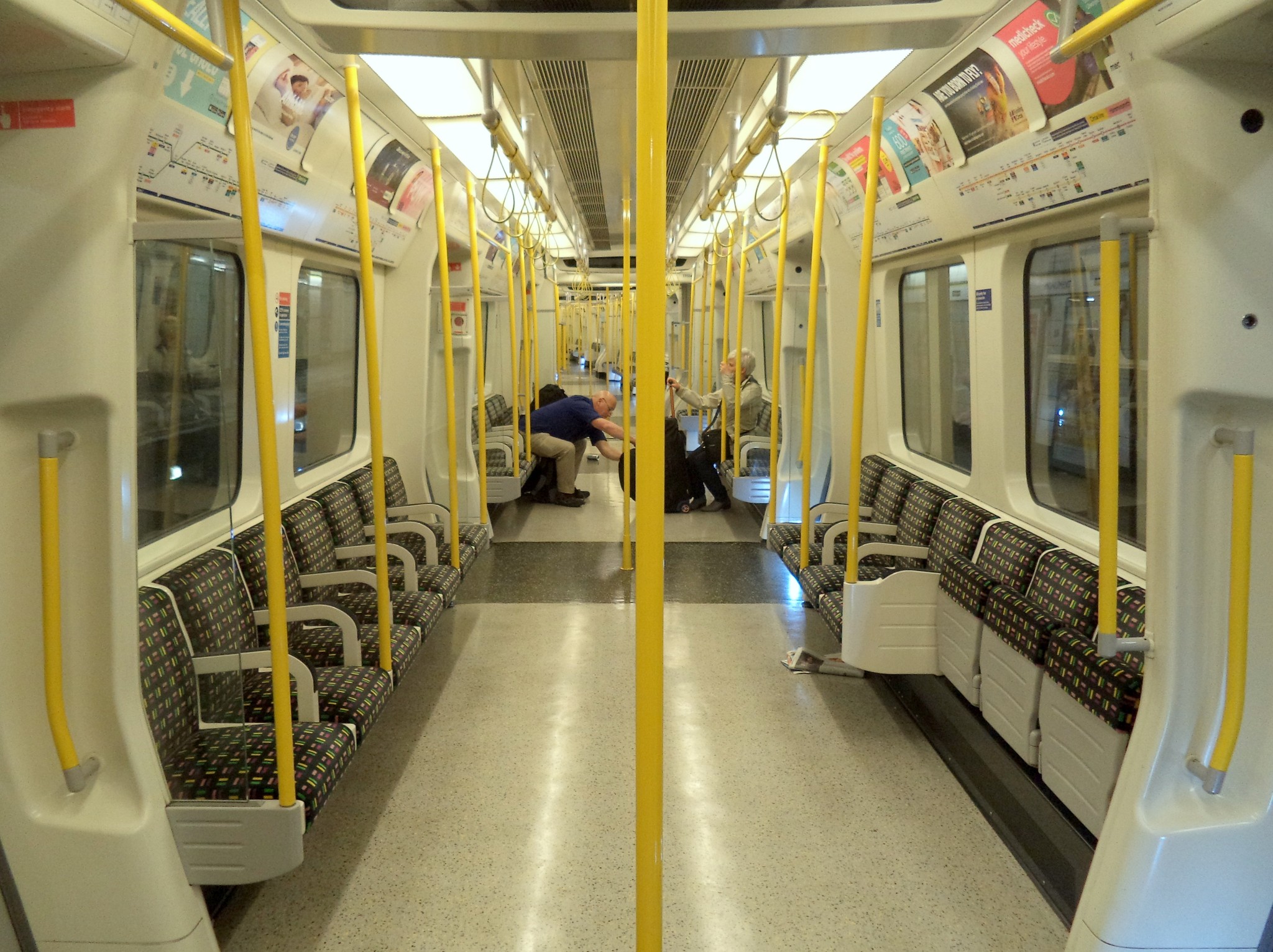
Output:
[703,429,732,462]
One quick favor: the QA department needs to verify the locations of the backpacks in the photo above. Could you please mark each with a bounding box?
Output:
[530,384,569,413]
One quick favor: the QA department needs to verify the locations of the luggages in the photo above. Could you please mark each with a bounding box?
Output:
[619,378,691,513]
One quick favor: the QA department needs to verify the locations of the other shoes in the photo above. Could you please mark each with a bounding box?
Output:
[701,500,731,512]
[556,490,585,507]
[573,488,590,499]
[690,492,706,510]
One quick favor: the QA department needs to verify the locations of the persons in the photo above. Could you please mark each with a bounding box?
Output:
[517,390,635,507]
[666,348,762,512]
[274,70,345,131]
[1072,5,1115,90]
[976,64,1013,142]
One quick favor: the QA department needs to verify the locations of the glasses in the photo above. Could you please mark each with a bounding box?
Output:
[599,398,613,414]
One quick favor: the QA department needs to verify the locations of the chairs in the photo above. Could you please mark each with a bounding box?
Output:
[573,338,638,387]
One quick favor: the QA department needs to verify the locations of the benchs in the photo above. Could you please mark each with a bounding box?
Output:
[135,392,538,885]
[698,381,1143,842]
